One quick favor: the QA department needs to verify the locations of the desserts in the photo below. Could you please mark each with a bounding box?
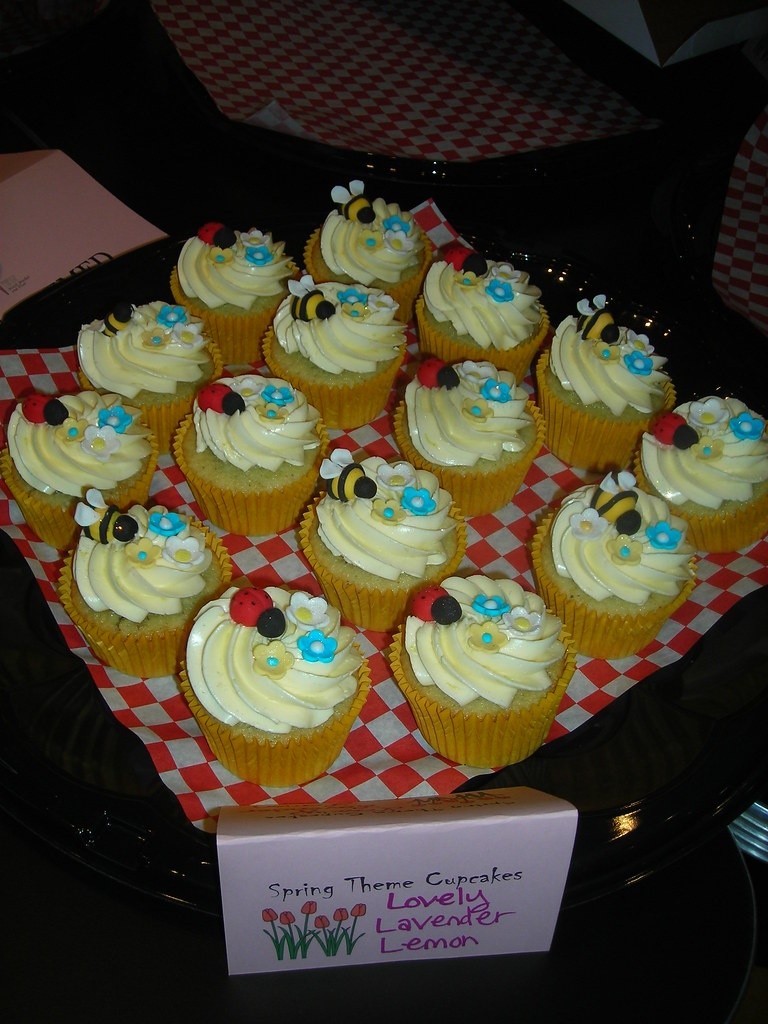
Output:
[7,174,766,786]
